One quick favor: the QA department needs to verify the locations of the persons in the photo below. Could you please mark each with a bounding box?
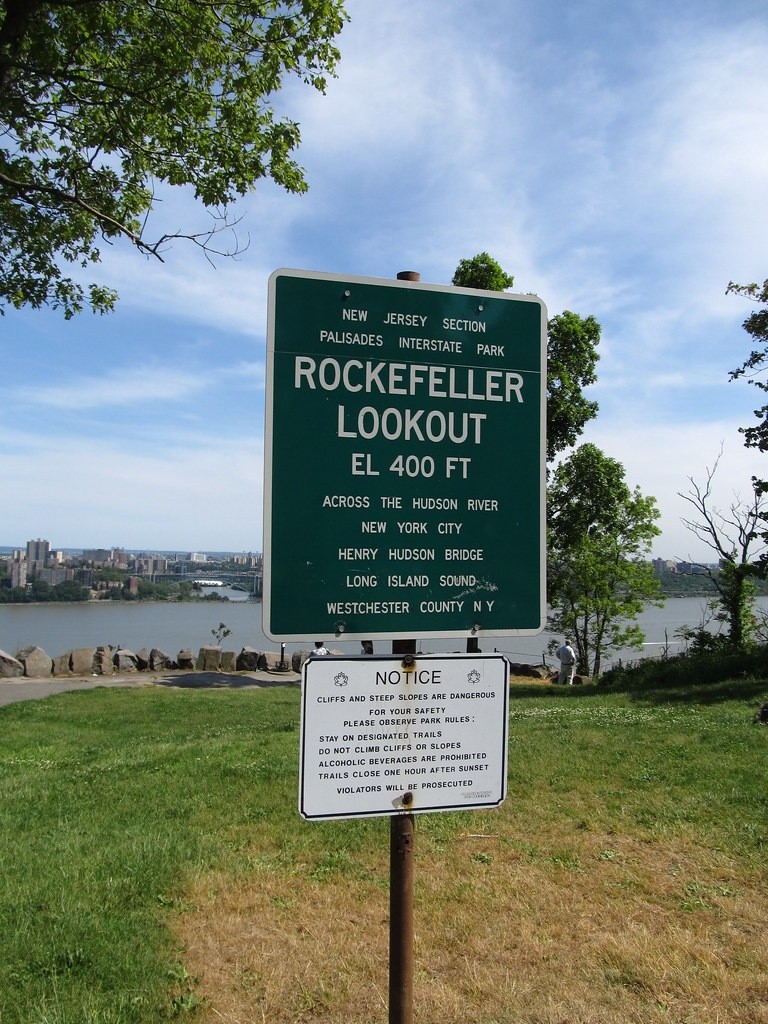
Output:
[556,640,577,686]
[309,642,331,658]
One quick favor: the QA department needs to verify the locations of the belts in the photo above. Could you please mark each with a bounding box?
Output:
[561,663,572,665]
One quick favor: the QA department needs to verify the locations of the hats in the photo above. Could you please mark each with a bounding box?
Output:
[566,640,571,643]
